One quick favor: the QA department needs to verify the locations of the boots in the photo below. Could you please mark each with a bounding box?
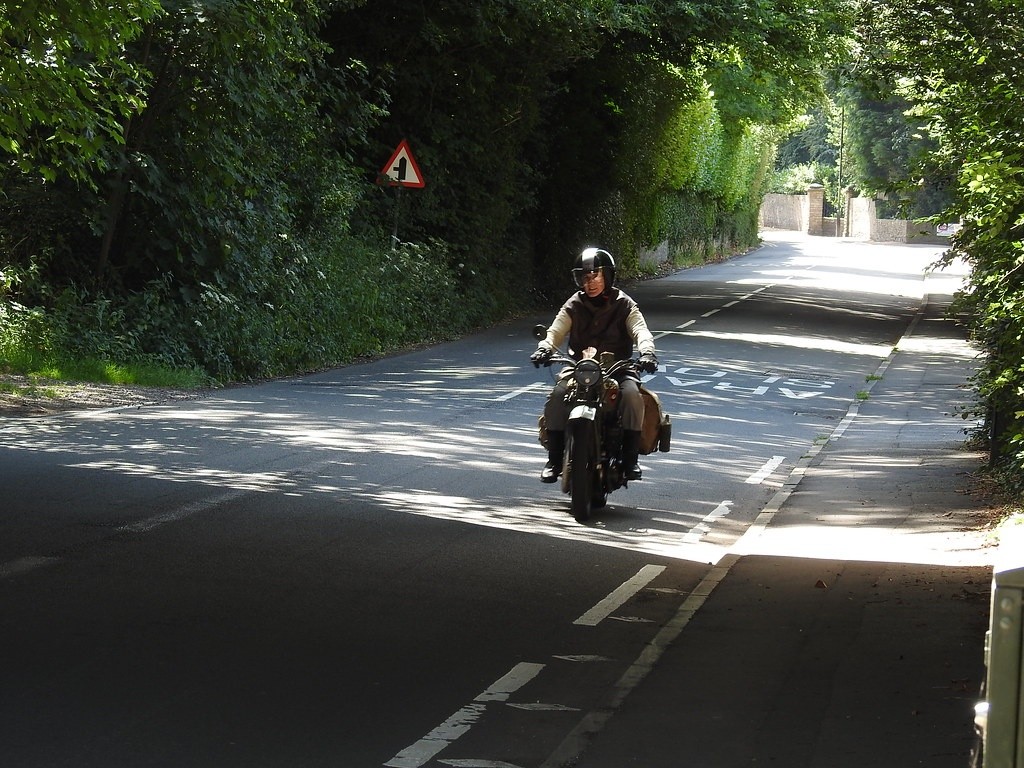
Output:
[622,429,642,480]
[539,429,564,483]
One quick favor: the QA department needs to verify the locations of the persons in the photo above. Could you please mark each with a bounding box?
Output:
[528,248,660,483]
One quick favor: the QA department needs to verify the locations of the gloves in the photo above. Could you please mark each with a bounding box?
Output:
[635,354,659,375]
[529,347,553,369]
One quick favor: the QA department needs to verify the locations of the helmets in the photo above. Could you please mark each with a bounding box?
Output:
[572,248,616,293]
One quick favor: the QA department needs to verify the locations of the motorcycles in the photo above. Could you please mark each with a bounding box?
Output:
[528,323,659,523]
